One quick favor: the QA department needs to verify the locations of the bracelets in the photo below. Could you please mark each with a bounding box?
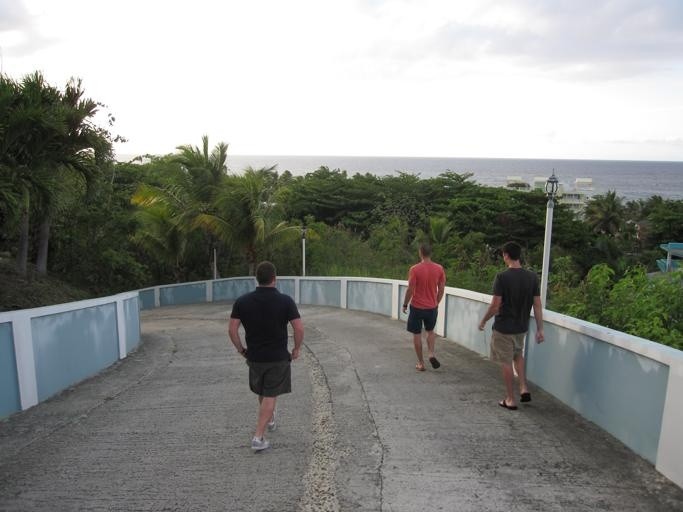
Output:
[402,304,407,307]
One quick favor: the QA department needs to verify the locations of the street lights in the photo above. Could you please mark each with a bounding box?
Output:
[299,225,307,277]
[538,166,560,307]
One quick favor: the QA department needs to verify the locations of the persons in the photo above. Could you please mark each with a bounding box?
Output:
[227,260,306,451]
[477,240,546,410]
[401,244,446,372]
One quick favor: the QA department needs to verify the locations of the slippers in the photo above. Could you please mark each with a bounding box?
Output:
[499,399,517,410]
[430,357,441,369]
[416,364,425,370]
[520,393,531,402]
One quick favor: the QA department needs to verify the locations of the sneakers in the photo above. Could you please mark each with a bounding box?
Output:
[268,413,276,431]
[252,436,268,450]
